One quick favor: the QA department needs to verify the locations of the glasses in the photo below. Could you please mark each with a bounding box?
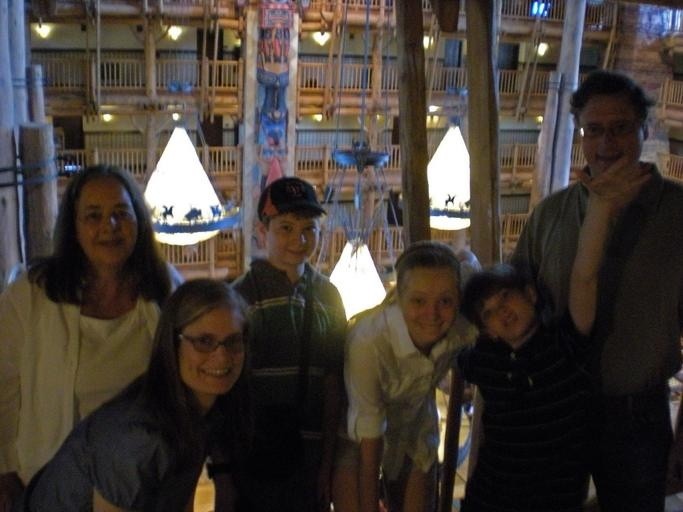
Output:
[578,119,645,142]
[178,332,250,354]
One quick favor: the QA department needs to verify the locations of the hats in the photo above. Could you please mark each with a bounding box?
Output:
[257,177,327,224]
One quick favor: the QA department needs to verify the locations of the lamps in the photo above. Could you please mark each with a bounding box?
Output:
[142,119,225,249]
[310,0,407,323]
[425,52,471,231]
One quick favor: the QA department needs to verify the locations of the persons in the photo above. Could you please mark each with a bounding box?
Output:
[333,242,479,512]
[204,178,349,511]
[457,260,594,512]
[16,280,252,512]
[0,161,184,512]
[513,71,683,512]
